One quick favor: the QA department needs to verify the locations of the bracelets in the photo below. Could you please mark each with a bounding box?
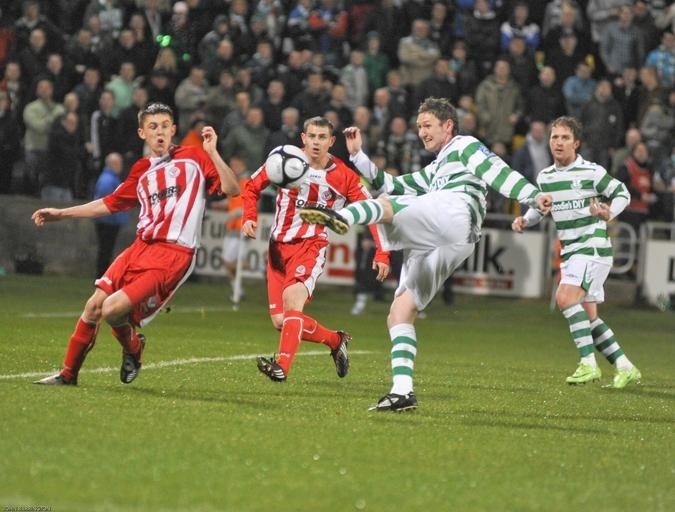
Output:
[352,148,360,157]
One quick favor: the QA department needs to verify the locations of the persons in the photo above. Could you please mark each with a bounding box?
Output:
[91,149,126,286]
[240,115,391,385]
[511,115,642,390]
[295,94,554,411]
[29,101,244,386]
[2,0,674,218]
[221,155,255,308]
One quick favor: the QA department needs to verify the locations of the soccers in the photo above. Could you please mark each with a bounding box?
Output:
[265,145,311,189]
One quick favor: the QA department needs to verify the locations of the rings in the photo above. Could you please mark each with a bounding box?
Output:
[514,218,519,225]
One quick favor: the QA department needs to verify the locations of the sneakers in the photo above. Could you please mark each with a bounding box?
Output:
[31,373,80,387]
[119,332,146,384]
[604,367,644,388]
[329,327,353,378]
[366,390,419,414]
[253,354,287,383]
[298,206,350,236]
[564,362,603,386]
